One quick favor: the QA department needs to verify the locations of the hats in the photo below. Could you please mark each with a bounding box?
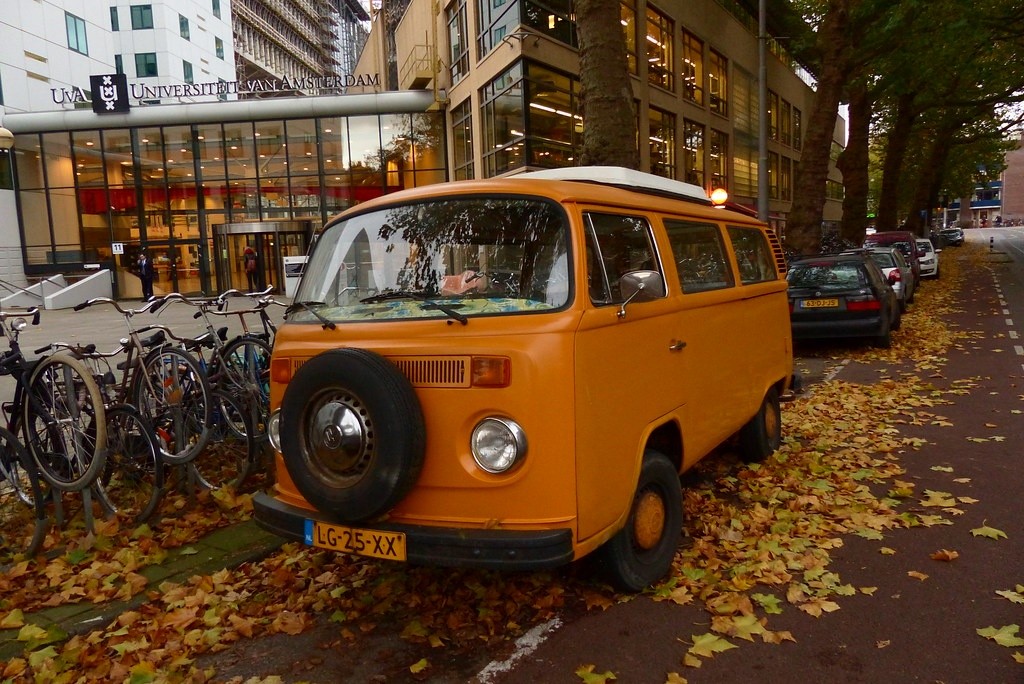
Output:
[244,248,253,254]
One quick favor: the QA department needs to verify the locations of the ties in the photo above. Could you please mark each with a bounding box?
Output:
[142,260,145,266]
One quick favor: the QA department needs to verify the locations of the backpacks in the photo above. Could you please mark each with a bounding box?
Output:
[246,254,256,273]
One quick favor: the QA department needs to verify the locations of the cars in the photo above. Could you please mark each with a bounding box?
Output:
[785,231,942,348]
[939,228,965,246]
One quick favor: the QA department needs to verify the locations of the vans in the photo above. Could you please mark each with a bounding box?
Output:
[252,163,796,593]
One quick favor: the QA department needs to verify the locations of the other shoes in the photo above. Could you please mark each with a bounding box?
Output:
[141,298,148,302]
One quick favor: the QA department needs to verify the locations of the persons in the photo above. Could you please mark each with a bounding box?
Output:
[137,253,155,304]
[981,214,986,227]
[243,247,262,297]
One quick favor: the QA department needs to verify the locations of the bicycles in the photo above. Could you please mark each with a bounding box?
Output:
[0,284,290,558]
